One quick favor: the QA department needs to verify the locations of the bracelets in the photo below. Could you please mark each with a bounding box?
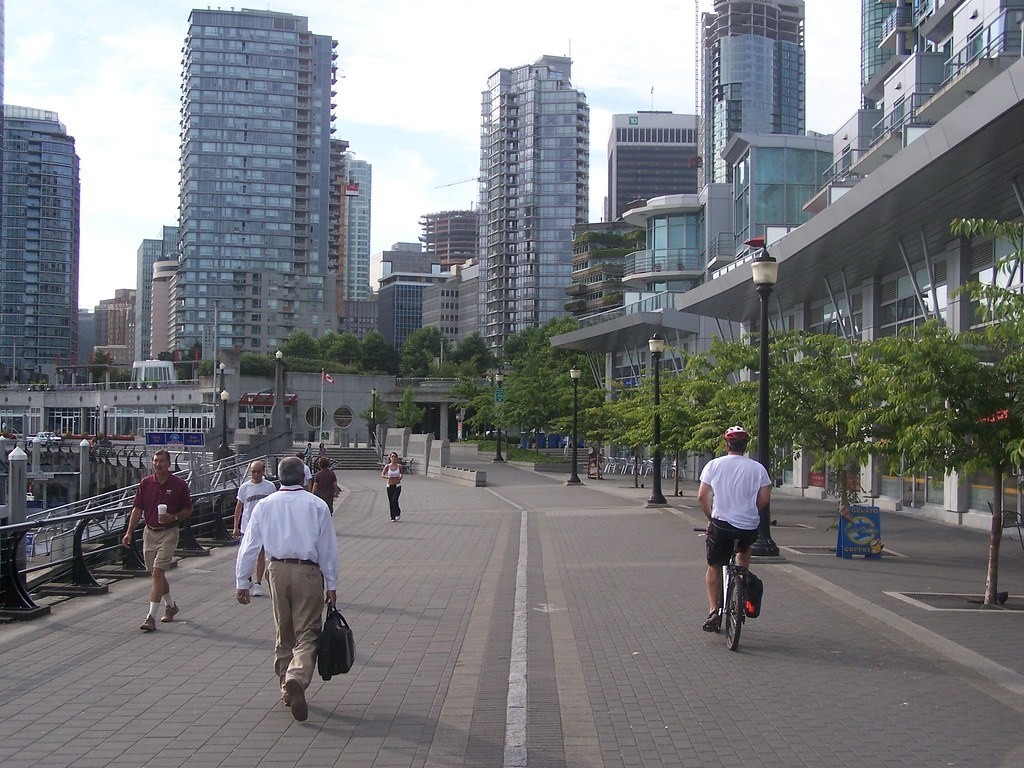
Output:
[173,513,177,521]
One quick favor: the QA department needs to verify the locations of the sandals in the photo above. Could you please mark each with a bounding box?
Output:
[703,611,721,632]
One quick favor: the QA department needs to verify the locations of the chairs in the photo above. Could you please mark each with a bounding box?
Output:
[603,457,686,480]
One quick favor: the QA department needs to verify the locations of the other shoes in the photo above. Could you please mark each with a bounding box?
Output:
[280,677,291,707]
[286,680,308,721]
[391,515,400,521]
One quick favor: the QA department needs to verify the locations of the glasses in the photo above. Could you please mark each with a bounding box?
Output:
[250,470,262,474]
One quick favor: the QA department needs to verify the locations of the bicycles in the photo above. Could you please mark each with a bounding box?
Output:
[693,527,750,651]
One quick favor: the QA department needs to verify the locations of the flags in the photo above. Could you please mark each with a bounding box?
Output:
[324,371,336,384]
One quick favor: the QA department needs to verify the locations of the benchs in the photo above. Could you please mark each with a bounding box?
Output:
[377,455,413,473]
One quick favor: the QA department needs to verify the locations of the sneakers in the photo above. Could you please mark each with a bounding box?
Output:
[139,615,156,632]
[160,604,179,622]
[253,583,263,596]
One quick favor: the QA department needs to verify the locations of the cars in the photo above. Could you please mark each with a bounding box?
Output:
[26,431,62,441]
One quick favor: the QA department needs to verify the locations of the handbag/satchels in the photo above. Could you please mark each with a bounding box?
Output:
[736,566,763,618]
[318,602,355,681]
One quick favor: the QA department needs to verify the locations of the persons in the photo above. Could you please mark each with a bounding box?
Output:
[312,457,338,518]
[2,420,7,433]
[318,443,327,457]
[382,452,403,522]
[123,450,192,631]
[698,426,770,632]
[235,456,339,721]
[304,443,312,467]
[294,452,313,495]
[233,461,277,596]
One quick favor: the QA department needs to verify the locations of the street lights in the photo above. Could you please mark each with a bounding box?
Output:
[749,248,788,564]
[170,403,176,432]
[95,405,100,444]
[219,363,226,402]
[103,404,110,440]
[440,337,445,367]
[564,364,585,486]
[371,386,377,447]
[492,371,508,463]
[217,389,230,459]
[643,332,672,509]
[270,350,286,428]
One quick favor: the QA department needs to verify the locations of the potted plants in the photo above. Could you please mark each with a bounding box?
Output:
[139,378,148,389]
[152,378,159,389]
[39,380,47,390]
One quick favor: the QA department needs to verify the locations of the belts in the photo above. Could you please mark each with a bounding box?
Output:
[271,556,319,567]
[147,524,178,532]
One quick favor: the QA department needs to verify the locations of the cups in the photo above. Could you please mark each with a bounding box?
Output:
[157,504,168,523]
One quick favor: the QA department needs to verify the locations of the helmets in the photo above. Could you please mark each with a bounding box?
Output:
[724,426,750,441]
